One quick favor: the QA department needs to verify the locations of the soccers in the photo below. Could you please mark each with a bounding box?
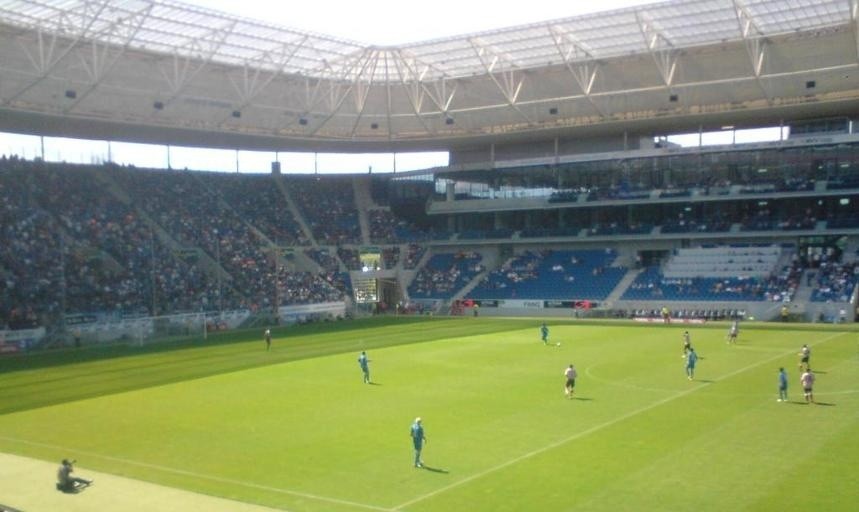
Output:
[557,342,560,346]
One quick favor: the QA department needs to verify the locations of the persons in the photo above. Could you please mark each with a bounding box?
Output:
[357,351,370,383]
[685,347,698,380]
[776,366,789,403]
[409,416,427,468]
[797,343,811,371]
[263,329,271,350]
[681,331,691,358]
[799,368,815,404]
[727,320,740,343]
[563,363,578,400]
[1,149,859,332]
[56,458,93,491]
[540,322,550,344]
